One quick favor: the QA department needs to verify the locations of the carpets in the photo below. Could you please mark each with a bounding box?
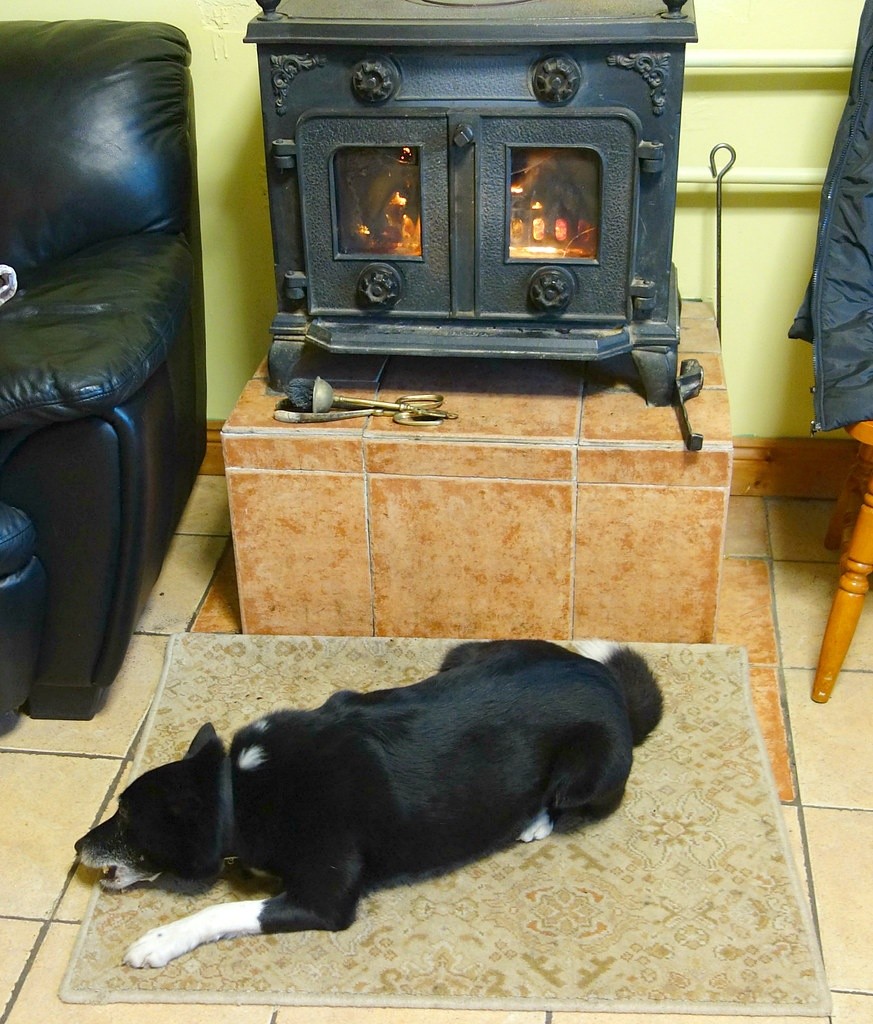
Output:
[59,628,835,1019]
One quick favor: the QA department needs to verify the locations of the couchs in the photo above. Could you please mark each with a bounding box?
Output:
[0,17,208,744]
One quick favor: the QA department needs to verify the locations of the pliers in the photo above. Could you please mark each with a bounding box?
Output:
[273,394,445,428]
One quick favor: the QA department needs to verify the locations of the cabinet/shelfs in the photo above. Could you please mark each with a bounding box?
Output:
[242,1,708,456]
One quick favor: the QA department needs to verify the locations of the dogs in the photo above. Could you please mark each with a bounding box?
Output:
[74,636,664,970]
